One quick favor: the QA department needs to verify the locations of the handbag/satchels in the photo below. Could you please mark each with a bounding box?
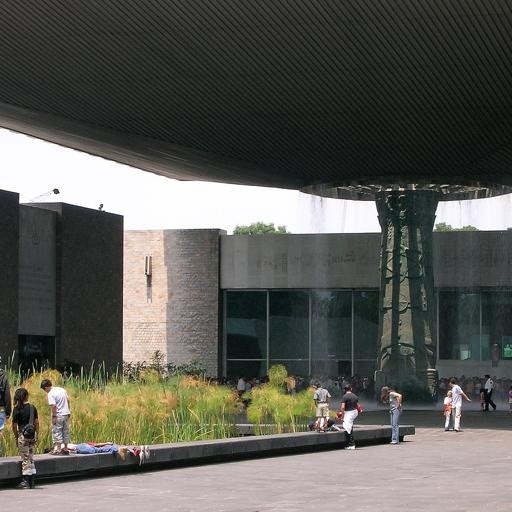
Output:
[22,424,35,439]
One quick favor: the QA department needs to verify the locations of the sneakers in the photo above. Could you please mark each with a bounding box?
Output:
[484,405,496,411]
[445,427,459,431]
[344,445,355,449]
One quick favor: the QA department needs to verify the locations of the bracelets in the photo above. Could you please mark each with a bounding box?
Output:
[53,415,57,417]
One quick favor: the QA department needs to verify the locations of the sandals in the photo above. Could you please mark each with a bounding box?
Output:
[49,449,69,455]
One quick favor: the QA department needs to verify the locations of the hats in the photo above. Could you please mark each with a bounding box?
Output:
[484,375,489,377]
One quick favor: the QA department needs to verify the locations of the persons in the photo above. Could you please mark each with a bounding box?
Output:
[12,387,45,488]
[313,384,332,432]
[43,441,128,462]
[40,377,72,454]
[484,375,498,410]
[206,375,270,404]
[437,374,511,398]
[0,368,12,432]
[450,378,471,432]
[306,375,369,393]
[309,415,345,432]
[341,382,360,450]
[380,382,404,445]
[479,387,485,412]
[444,392,452,431]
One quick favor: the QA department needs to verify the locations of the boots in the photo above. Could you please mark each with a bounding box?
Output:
[14,474,35,489]
